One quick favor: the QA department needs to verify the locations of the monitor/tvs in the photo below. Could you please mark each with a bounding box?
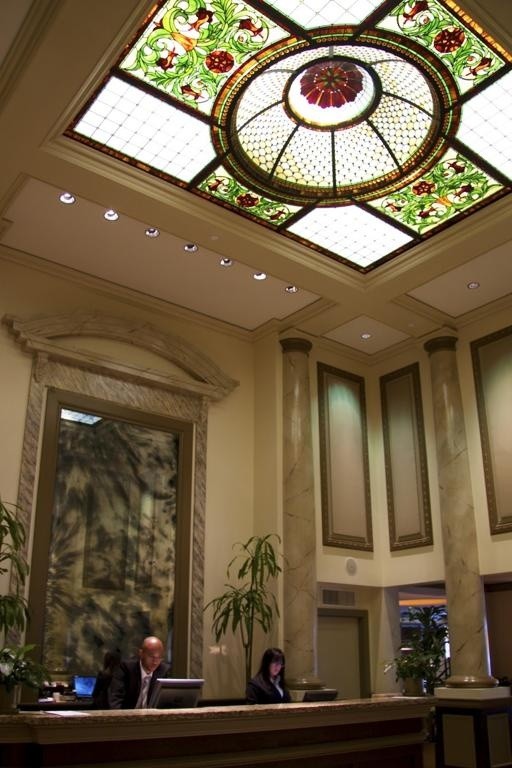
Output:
[147,678,205,709]
[302,690,337,702]
[72,675,98,698]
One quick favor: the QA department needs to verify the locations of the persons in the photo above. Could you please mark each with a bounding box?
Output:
[244,647,291,704]
[91,648,121,709]
[109,636,174,710]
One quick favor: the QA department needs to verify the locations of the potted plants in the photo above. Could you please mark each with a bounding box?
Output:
[383,603,448,694]
[0,643,51,711]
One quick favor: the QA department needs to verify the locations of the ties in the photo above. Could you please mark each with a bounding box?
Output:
[138,678,150,708]
